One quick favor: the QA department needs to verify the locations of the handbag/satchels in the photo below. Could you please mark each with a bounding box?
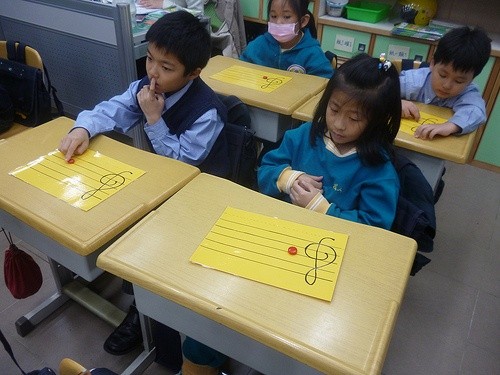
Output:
[0,86,16,133]
[2,227,42,299]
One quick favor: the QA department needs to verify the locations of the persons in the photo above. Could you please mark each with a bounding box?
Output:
[58,12,230,353]
[398,25,493,139]
[238,0,335,78]
[137,0,209,11]
[181,53,401,375]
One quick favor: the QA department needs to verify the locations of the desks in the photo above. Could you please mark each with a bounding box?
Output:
[132,9,212,61]
[291,87,479,201]
[0,115,201,375]
[199,54,331,151]
[95,173,418,375]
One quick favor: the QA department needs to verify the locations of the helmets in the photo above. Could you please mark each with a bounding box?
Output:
[399,0,437,27]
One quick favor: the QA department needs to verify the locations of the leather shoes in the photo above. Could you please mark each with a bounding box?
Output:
[103,304,143,356]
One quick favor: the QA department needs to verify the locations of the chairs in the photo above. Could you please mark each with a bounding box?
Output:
[377,51,433,75]
[0,41,50,141]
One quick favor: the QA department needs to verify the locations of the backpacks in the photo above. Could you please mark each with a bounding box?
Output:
[390,153,436,275]
[213,93,259,193]
[0,39,64,127]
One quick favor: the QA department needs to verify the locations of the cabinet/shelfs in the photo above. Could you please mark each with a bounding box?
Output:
[238,0,500,175]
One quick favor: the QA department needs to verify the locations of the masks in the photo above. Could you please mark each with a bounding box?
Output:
[267,21,301,43]
